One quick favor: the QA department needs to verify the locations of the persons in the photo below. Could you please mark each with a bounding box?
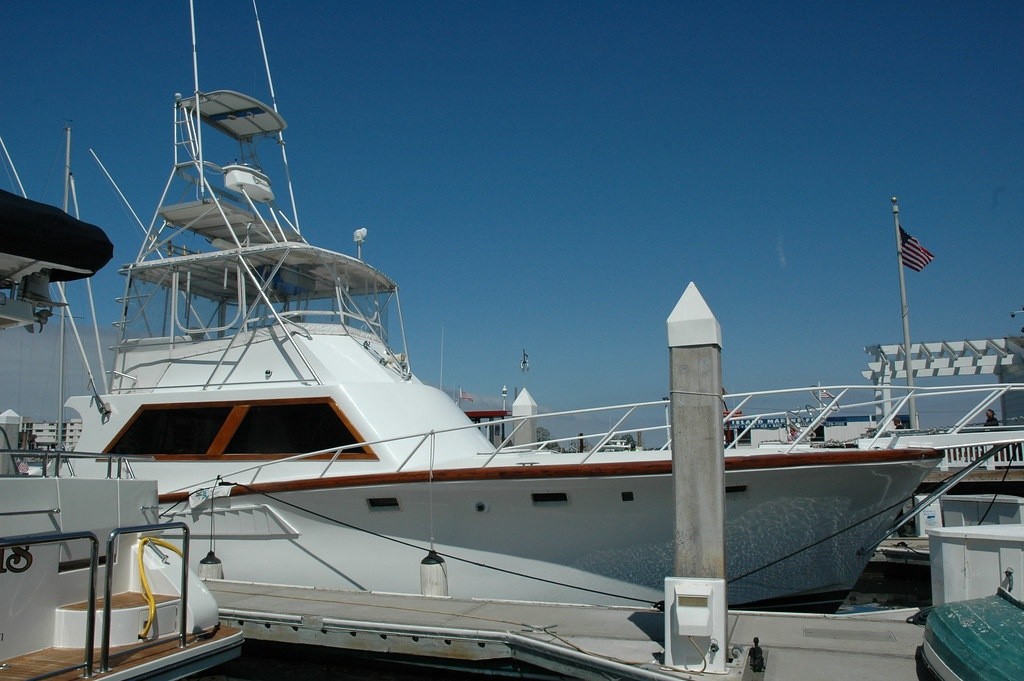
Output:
[894,418,903,429]
[984,409,999,426]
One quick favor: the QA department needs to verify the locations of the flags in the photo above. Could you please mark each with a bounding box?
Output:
[462,392,473,401]
[899,226,934,273]
[821,390,833,398]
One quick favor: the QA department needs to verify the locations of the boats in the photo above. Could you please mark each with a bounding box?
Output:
[0,0,1023,681]
